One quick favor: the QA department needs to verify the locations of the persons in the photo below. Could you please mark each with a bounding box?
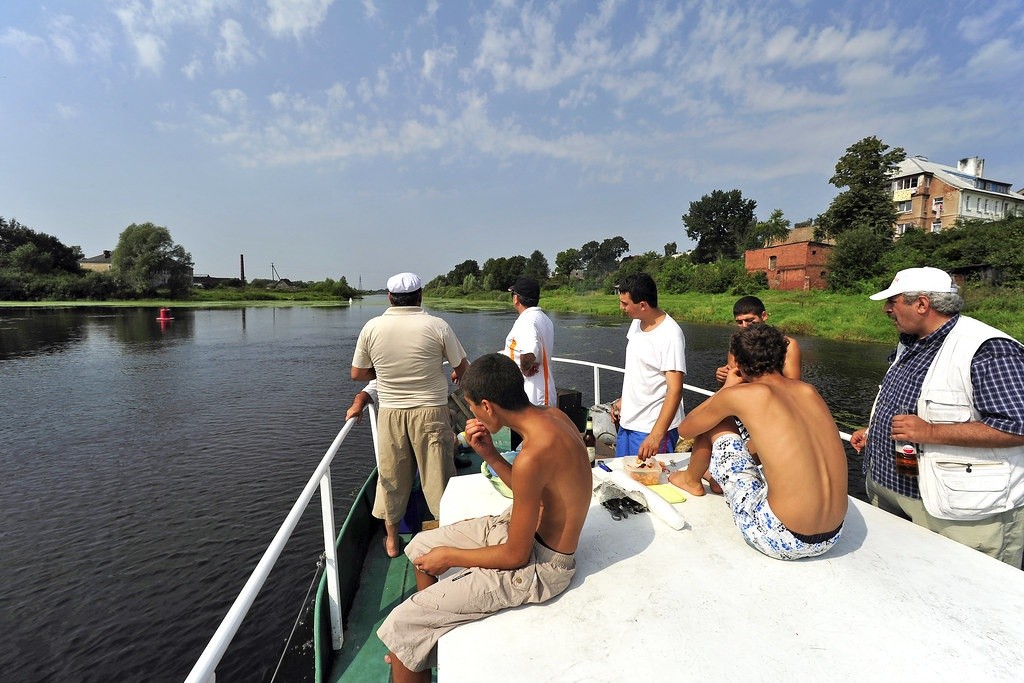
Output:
[713,293,804,440]
[851,266,1024,574]
[491,273,560,451]
[344,377,473,487]
[665,322,849,559]
[373,347,596,683]
[603,274,689,461]
[350,269,472,561]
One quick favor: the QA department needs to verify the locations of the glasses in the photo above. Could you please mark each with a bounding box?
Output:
[511,292,519,297]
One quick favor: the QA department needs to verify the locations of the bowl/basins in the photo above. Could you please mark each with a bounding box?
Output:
[481,451,520,499]
[622,455,662,486]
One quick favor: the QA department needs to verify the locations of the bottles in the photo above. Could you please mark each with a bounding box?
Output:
[895,405,919,476]
[583,417,596,468]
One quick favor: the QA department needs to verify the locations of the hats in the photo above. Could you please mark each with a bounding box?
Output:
[508,277,540,297]
[869,266,957,302]
[387,272,421,294]
